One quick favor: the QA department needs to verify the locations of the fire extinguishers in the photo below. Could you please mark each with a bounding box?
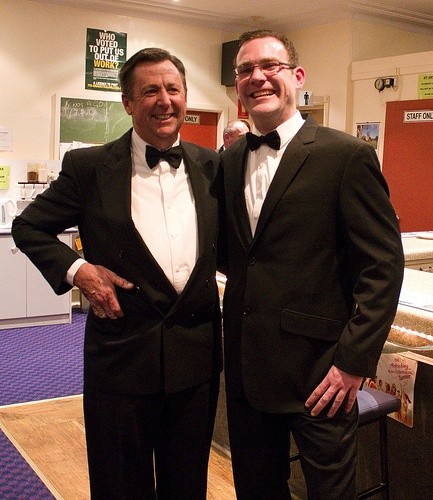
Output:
[237,96,249,119]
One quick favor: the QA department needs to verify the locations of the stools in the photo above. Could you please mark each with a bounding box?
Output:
[290,384,400,499]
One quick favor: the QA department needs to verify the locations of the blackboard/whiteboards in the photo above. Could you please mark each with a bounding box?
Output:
[52,95,133,165]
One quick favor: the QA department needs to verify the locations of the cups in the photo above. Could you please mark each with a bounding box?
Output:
[26,159,59,183]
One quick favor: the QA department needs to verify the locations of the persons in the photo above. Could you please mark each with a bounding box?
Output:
[11,48,222,500]
[217,120,250,155]
[218,30,406,500]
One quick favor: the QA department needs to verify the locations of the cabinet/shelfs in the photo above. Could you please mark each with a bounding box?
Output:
[0,234,78,330]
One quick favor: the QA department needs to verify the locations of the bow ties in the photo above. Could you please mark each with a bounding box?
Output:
[244,129,281,152]
[145,144,183,169]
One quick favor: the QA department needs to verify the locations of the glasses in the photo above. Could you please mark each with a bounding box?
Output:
[233,60,295,77]
